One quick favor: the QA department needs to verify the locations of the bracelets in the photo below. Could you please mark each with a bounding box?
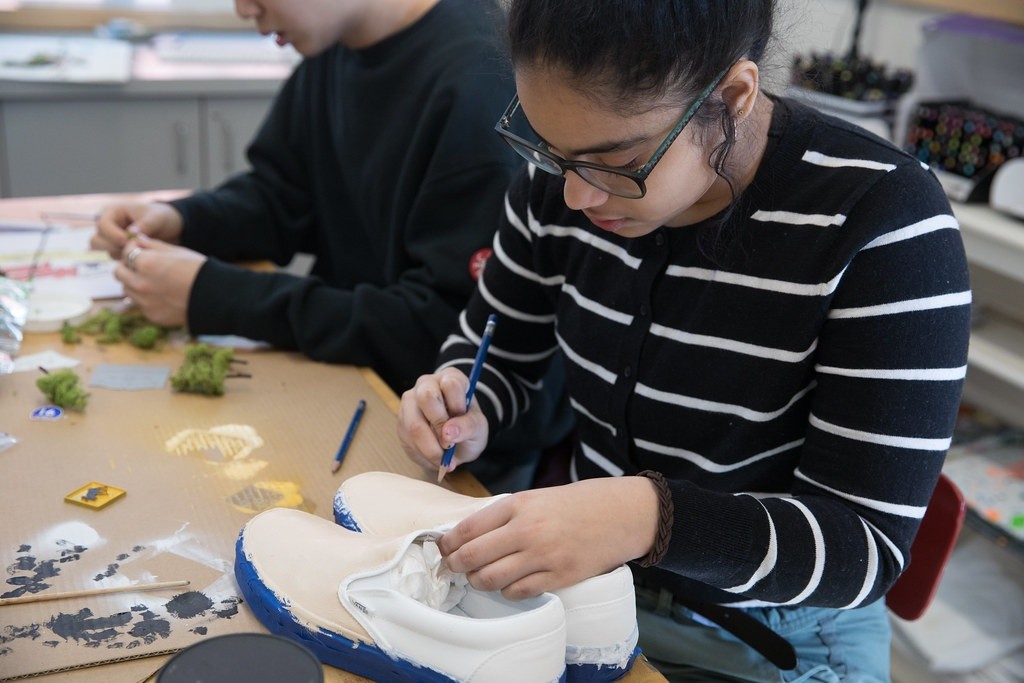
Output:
[636,469,673,567]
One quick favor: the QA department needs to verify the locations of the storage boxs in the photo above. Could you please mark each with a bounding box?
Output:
[902,99,1024,201]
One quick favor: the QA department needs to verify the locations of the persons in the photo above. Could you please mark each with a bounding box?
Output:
[91,0,538,395]
[397,1,972,683]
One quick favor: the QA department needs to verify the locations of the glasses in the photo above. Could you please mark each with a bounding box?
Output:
[495,47,748,199]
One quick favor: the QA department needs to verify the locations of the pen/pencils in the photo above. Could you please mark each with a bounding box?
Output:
[436,314,497,484]
[331,399,366,473]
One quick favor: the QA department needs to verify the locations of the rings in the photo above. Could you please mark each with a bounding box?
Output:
[128,247,142,269]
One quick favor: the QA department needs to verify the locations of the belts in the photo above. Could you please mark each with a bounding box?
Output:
[631,563,797,671]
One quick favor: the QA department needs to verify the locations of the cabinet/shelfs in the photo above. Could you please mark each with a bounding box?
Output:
[0,35,308,192]
[940,196,1024,560]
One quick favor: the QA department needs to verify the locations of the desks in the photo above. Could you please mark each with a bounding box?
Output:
[0,194,672,683]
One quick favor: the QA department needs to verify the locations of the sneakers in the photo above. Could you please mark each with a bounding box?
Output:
[234,507,567,683]
[333,471,642,683]
